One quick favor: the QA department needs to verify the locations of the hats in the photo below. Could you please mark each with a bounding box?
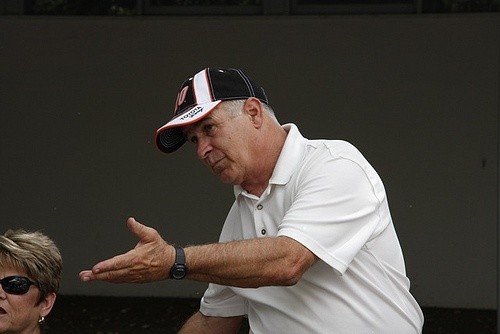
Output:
[154,66,268,154]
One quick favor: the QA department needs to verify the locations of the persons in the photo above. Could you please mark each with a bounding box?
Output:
[0,229,63,334]
[79,67,425,334]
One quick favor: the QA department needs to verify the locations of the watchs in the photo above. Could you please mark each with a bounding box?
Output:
[169,244,189,279]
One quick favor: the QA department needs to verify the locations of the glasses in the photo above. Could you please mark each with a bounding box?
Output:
[0,275,35,295]
